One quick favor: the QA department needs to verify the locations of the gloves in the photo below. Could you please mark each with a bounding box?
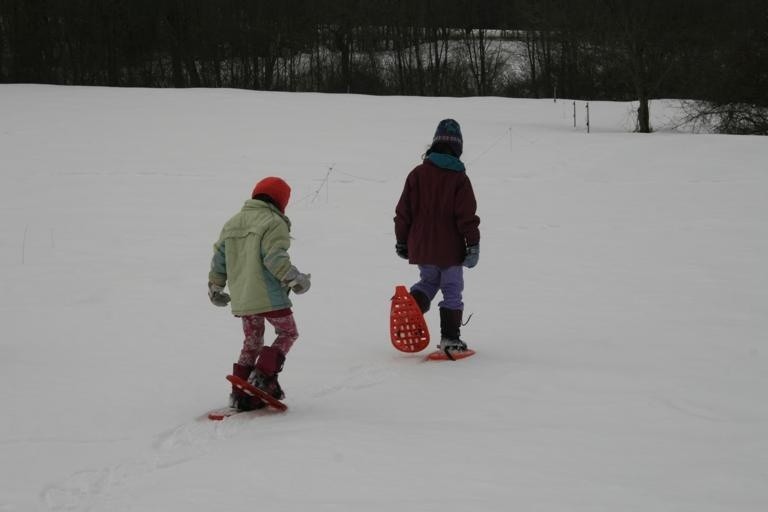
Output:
[463,247,480,268]
[395,245,408,259]
[281,265,311,294]
[208,285,231,307]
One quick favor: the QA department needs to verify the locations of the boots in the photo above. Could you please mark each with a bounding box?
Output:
[439,307,467,353]
[232,346,287,410]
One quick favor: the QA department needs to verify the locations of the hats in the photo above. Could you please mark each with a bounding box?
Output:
[252,177,291,213]
[433,119,463,147]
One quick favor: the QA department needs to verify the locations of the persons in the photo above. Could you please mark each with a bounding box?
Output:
[207,177,311,410]
[393,119,481,352]
